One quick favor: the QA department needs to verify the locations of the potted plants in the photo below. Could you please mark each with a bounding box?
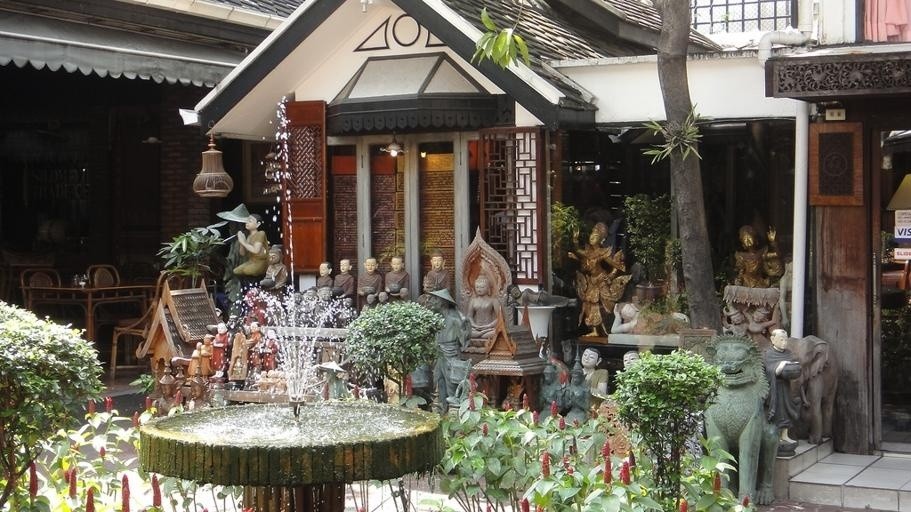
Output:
[622,192,672,303]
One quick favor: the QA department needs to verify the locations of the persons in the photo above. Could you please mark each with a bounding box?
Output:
[288,251,451,332]
[742,300,784,351]
[407,287,475,411]
[463,274,503,340]
[153,314,298,417]
[257,242,289,302]
[734,220,786,290]
[232,214,273,279]
[506,283,580,312]
[209,202,253,323]
[492,343,643,428]
[762,328,805,445]
[610,299,690,336]
[305,361,356,400]
[722,300,751,340]
[570,220,625,339]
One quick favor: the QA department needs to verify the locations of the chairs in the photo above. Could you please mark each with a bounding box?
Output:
[18,265,188,380]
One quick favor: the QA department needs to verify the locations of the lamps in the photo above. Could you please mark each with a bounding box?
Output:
[379,130,405,158]
